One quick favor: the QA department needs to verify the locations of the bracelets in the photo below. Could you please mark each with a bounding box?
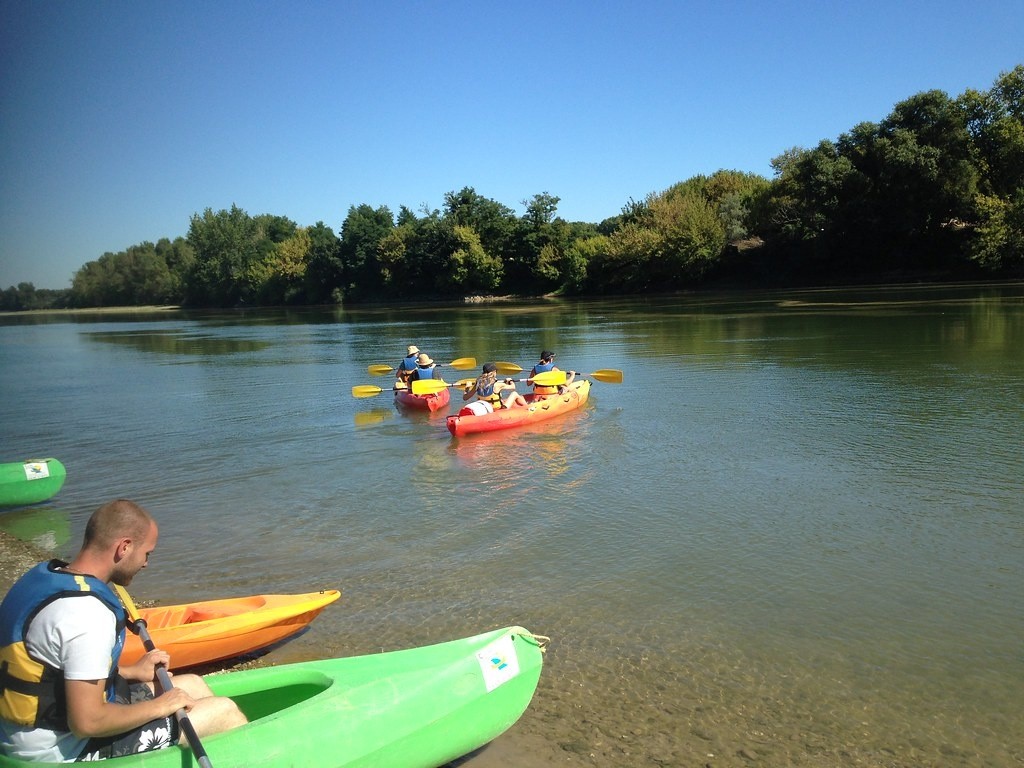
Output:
[508,380,513,384]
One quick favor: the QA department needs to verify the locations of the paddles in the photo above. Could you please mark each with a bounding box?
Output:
[367,357,477,375]
[352,378,477,398]
[412,371,567,394]
[108,583,215,767]
[494,359,623,384]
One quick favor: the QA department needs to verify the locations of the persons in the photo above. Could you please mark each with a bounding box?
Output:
[526,350,576,395]
[463,364,527,410]
[0,499,249,763]
[396,346,441,387]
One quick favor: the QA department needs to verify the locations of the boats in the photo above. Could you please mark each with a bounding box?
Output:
[0,457,68,506]
[0,508,73,552]
[446,379,592,438]
[117,589,341,673]
[393,384,450,412]
[0,622,551,768]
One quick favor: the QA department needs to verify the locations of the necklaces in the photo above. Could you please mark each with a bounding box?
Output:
[64,566,86,574]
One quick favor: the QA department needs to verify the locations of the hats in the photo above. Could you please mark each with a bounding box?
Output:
[407,346,420,357]
[541,352,554,361]
[483,363,498,373]
[415,354,433,365]
